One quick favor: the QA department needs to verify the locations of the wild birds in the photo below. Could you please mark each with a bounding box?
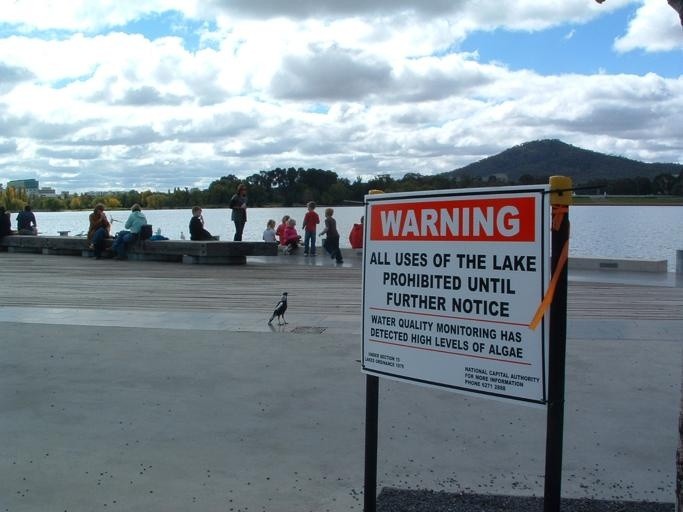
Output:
[266,291,289,325]
[109,214,118,224]
[180,231,186,240]
[76,230,85,238]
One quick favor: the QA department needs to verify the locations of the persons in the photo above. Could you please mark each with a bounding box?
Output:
[261,219,281,245]
[318,207,343,264]
[86,204,111,261]
[228,184,248,240]
[276,215,290,244]
[16,205,38,235]
[0,206,12,252]
[282,219,302,255]
[105,202,147,260]
[347,216,364,248]
[302,201,320,258]
[188,206,219,241]
